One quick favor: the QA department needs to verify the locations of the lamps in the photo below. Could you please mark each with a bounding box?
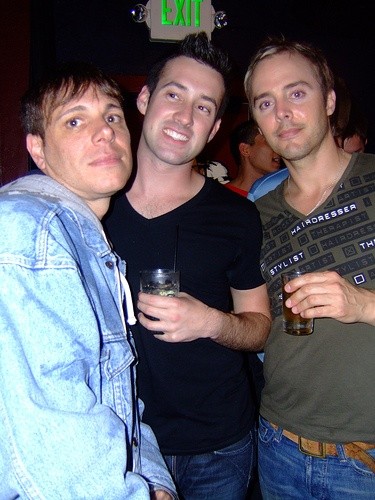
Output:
[130,0,227,43]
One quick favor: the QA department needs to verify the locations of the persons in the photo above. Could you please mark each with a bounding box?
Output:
[0,68,179,500]
[101,31,271,500]
[251,38,375,500]
[223,81,370,197]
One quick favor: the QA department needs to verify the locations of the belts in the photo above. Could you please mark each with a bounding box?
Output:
[269,422,375,473]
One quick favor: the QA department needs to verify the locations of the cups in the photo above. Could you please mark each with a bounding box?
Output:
[281,271,314,336]
[138,267,180,333]
